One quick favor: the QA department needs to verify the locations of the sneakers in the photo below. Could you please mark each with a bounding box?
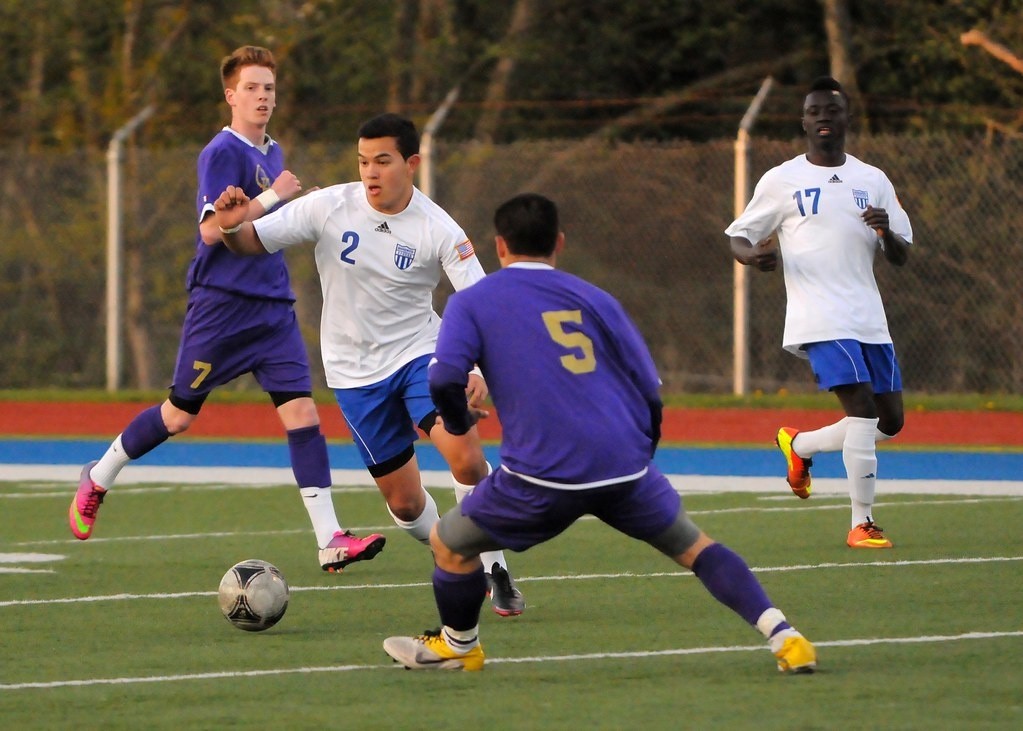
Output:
[318,529,386,574]
[846,516,893,548]
[774,636,818,672]
[484,561,526,617]
[382,627,486,671]
[70,461,108,540]
[774,426,813,499]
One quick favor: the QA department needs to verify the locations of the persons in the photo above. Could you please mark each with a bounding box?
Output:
[67,46,387,573]
[724,77,912,550]
[213,113,527,616]
[383,192,818,675]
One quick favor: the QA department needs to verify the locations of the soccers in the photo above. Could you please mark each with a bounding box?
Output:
[218,558,288,632]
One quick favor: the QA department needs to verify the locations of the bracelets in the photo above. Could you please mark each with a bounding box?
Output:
[219,224,242,233]
[255,188,280,212]
[468,366,484,380]
